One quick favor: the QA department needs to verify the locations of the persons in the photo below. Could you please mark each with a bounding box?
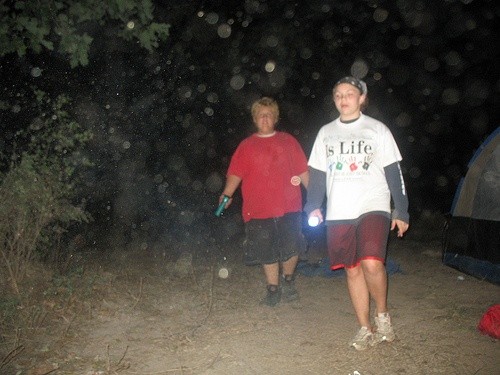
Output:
[304,77,411,351]
[217,95,310,308]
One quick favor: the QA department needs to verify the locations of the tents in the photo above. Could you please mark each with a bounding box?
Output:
[442,129,500,288]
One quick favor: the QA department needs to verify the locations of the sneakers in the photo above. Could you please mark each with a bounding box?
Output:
[258,284,282,308]
[349,325,374,350]
[279,274,298,302]
[372,311,394,343]
